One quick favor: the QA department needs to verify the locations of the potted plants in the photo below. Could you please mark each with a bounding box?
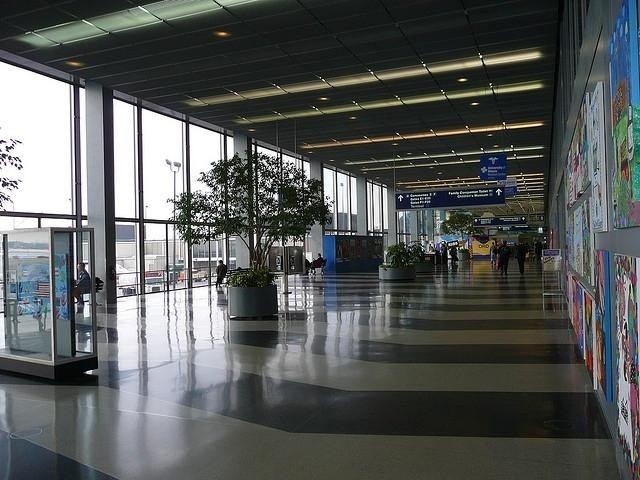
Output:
[441,213,485,261]
[377,240,433,280]
[164,146,336,320]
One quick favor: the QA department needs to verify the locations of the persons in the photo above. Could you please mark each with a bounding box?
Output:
[305,253,323,274]
[489,240,543,275]
[216,260,227,287]
[449,245,457,265]
[429,241,447,264]
[73,263,90,305]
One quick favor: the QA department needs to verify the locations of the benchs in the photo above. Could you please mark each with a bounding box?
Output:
[31,278,104,315]
